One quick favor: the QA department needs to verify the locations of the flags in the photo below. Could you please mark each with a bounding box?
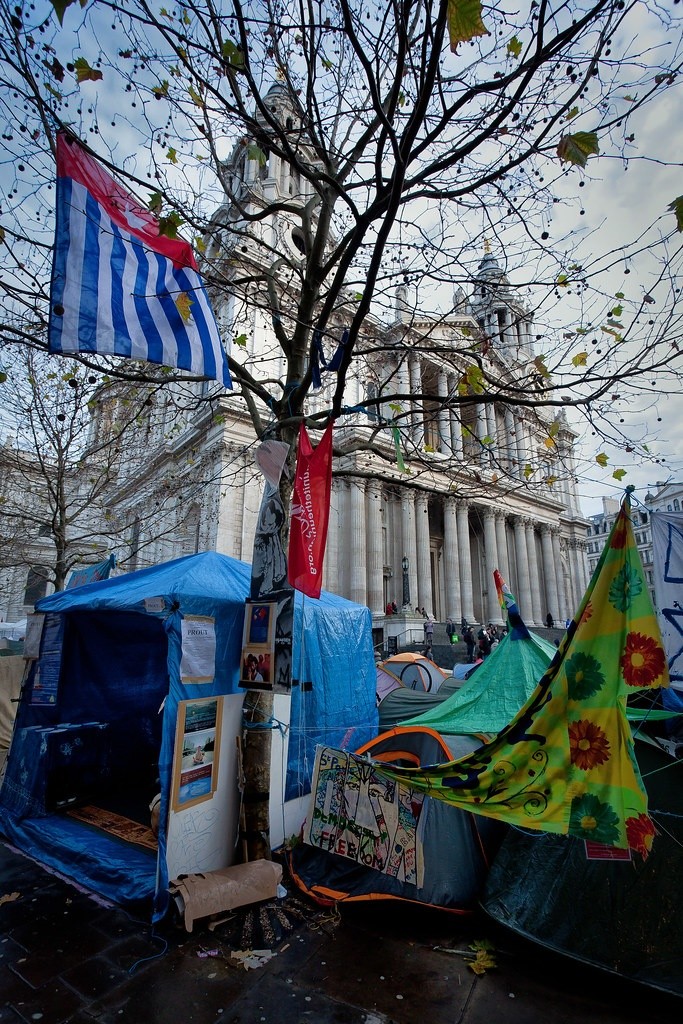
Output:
[45,128,237,392]
[285,428,336,601]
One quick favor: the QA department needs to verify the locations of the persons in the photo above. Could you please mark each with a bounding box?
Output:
[245,655,265,681]
[194,745,204,762]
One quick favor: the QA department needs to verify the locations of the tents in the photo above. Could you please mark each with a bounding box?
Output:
[4,483,681,1017]
